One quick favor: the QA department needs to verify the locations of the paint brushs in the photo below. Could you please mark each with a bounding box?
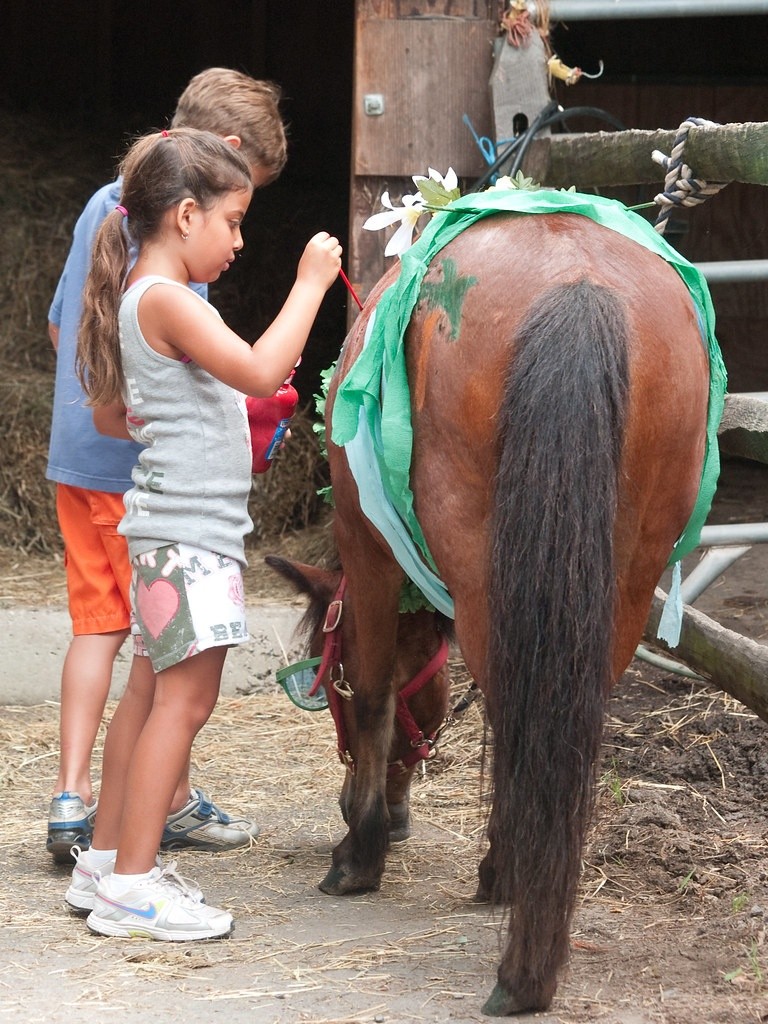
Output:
[337,265,364,313]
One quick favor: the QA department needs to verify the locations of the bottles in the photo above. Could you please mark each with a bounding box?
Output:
[244,351,306,472]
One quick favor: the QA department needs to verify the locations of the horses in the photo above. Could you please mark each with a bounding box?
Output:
[261,210,710,1019]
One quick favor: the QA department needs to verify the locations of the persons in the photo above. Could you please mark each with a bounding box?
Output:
[38,64,289,860]
[61,123,345,943]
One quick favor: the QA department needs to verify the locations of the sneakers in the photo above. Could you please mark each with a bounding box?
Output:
[63,845,205,912]
[156,789,258,852]
[46,792,100,856]
[87,868,235,942]
